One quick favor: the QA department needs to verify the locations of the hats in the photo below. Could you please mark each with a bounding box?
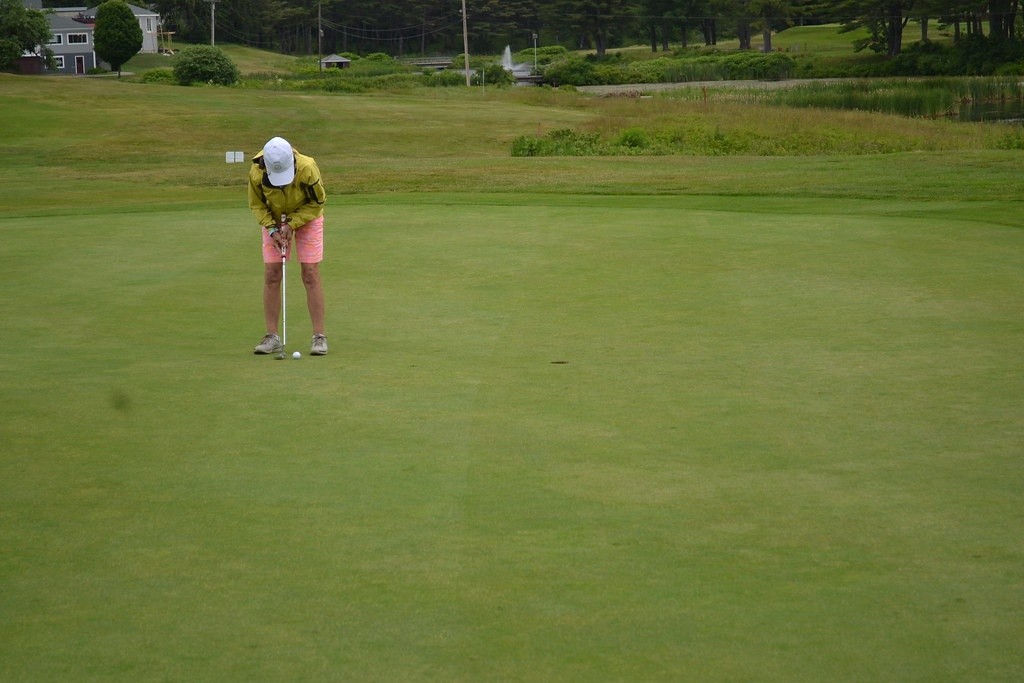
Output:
[263,136,295,186]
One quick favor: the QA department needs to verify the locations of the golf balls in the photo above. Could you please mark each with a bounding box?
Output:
[293,352,300,359]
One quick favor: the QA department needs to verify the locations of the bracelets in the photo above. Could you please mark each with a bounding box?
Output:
[269,228,279,237]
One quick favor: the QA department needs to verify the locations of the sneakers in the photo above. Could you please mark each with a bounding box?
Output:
[310,334,328,355]
[253,333,282,354]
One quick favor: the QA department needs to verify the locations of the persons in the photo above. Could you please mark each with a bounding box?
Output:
[247,136,329,356]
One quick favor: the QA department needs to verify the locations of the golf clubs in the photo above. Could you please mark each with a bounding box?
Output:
[275,213,287,360]
